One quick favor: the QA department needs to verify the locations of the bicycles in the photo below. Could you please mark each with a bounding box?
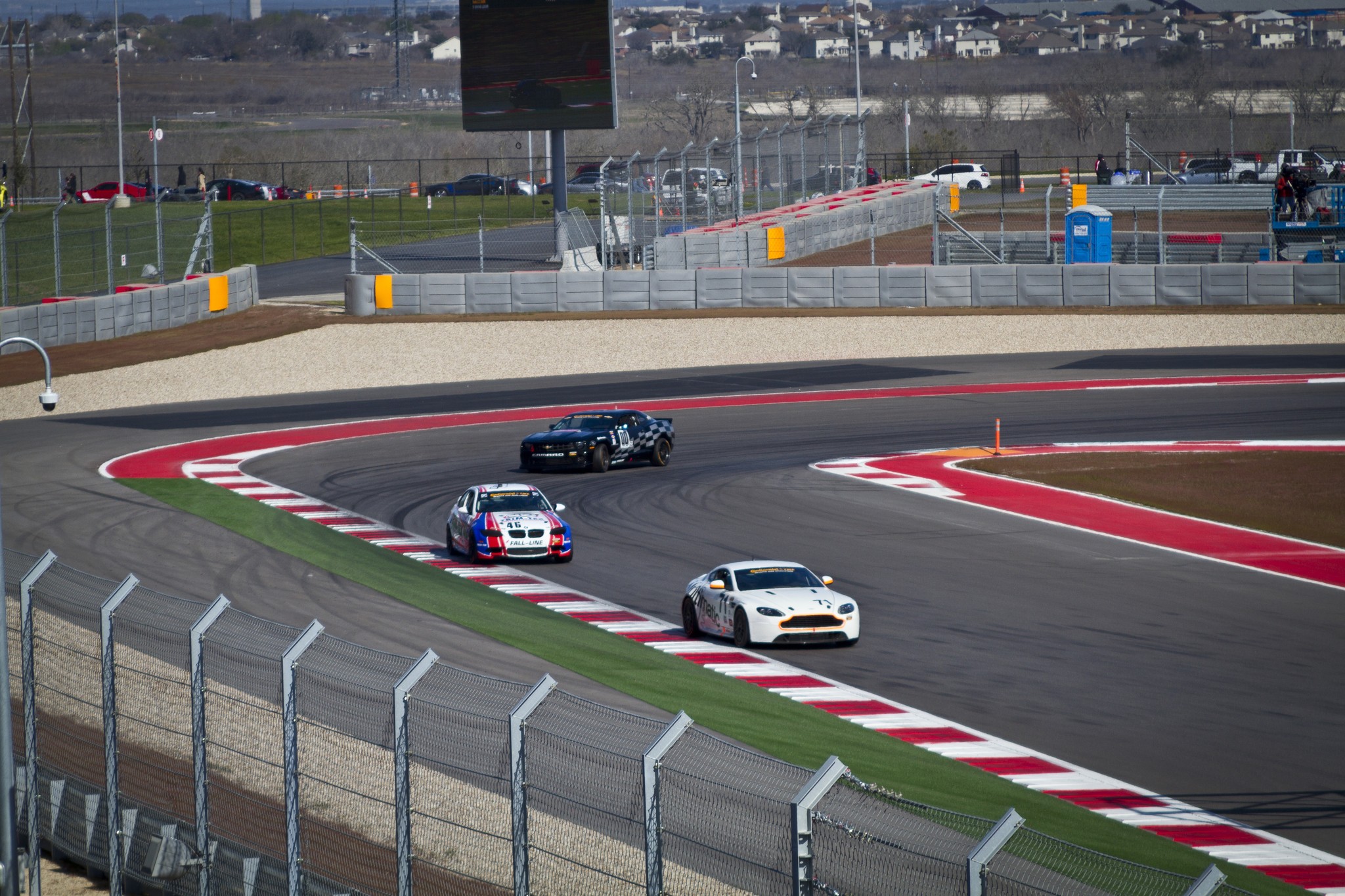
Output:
[884,166,914,183]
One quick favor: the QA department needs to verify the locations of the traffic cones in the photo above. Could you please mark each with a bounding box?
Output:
[1020,179,1026,193]
[268,191,272,201]
[742,182,744,192]
[674,199,679,215]
[649,183,653,191]
[317,190,321,199]
[1068,180,1071,187]
[9,196,14,206]
[363,189,368,198]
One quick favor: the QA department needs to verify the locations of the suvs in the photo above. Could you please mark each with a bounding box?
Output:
[787,167,882,192]
[1180,151,1262,173]
[909,163,992,190]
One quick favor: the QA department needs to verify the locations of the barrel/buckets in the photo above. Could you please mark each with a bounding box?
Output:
[1128,169,1142,183]
[1111,171,1127,185]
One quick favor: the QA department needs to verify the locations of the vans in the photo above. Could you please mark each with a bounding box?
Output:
[659,167,737,213]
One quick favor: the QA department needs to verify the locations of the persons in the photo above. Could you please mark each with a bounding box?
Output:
[60,173,78,204]
[1275,163,1297,213]
[760,159,775,191]
[1096,153,1110,185]
[144,165,206,202]
[680,166,698,206]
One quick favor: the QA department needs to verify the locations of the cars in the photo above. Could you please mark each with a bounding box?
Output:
[1159,164,1222,185]
[446,483,572,568]
[519,410,673,474]
[61,178,317,203]
[681,561,861,649]
[0,176,7,212]
[424,172,660,198]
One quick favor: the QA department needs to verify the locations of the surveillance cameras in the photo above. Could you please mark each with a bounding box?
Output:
[39,386,58,411]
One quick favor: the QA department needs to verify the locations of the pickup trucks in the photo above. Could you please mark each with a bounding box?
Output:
[1222,149,1345,184]
